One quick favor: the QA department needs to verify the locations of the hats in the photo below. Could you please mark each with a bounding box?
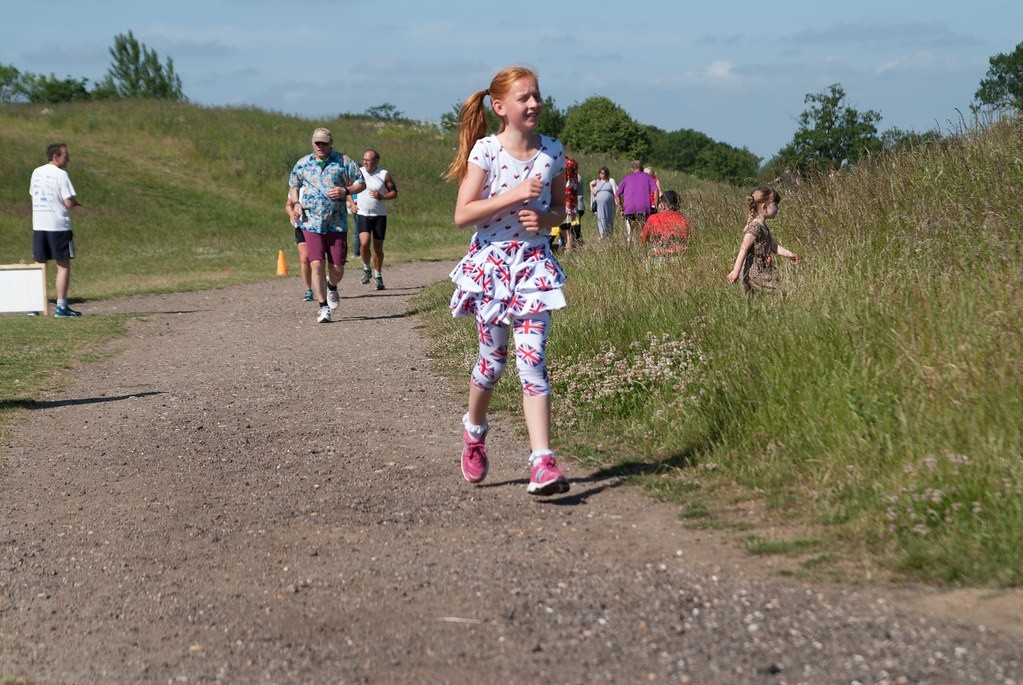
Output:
[661,190,679,211]
[311,127,332,144]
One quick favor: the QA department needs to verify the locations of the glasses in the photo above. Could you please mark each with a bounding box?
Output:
[314,142,329,146]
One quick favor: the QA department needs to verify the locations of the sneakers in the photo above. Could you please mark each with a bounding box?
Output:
[26,311,39,317]
[317,306,331,323]
[527,455,570,496]
[460,429,488,482]
[303,289,313,302]
[326,275,339,310]
[374,276,384,290]
[54,306,81,317]
[360,269,372,284]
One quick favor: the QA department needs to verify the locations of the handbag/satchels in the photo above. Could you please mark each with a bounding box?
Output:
[592,200,597,213]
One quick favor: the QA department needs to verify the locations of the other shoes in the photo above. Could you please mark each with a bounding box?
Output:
[353,251,360,258]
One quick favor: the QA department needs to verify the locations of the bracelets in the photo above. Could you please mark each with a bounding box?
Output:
[292,201,301,207]
[345,187,350,197]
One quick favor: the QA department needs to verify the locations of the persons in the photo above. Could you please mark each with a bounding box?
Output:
[453,67,571,496]
[589,166,620,241]
[616,160,663,244]
[825,164,842,181]
[285,127,397,323]
[640,190,692,278]
[536,154,586,254]
[27,142,82,317]
[765,166,802,187]
[726,188,797,301]
